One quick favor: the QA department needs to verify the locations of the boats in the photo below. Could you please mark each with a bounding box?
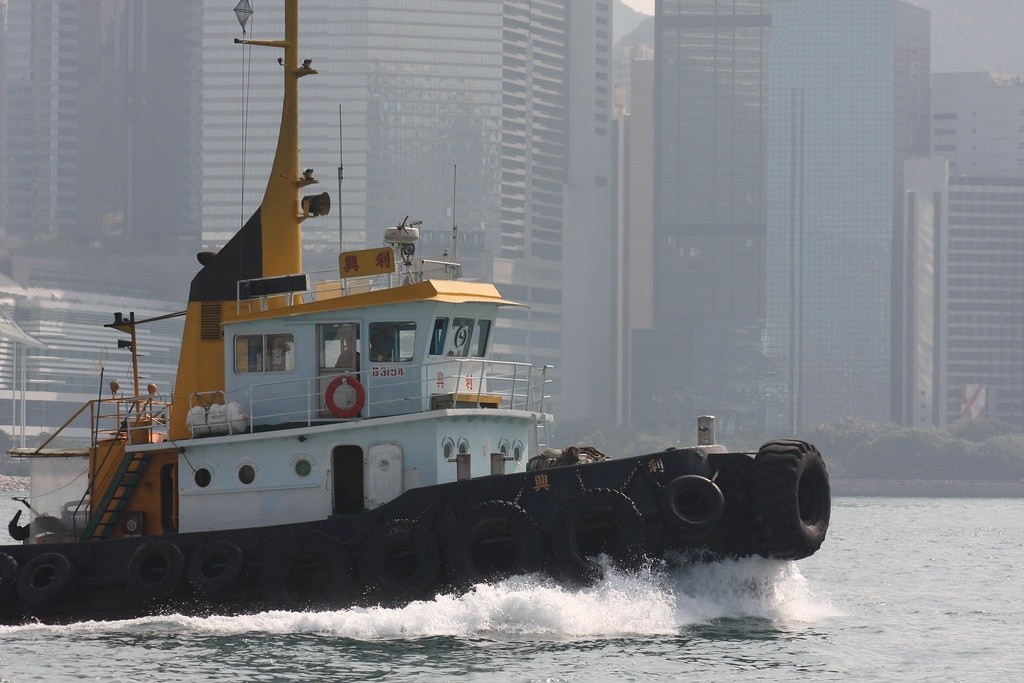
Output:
[0,0,832,626]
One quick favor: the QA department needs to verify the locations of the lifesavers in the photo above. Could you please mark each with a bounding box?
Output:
[189,540,244,594]
[659,475,724,532]
[361,518,441,595]
[551,488,648,577]
[16,552,74,607]
[447,498,547,589]
[325,375,365,417]
[264,529,351,613]
[748,437,832,562]
[0,552,18,606]
[127,538,187,600]
